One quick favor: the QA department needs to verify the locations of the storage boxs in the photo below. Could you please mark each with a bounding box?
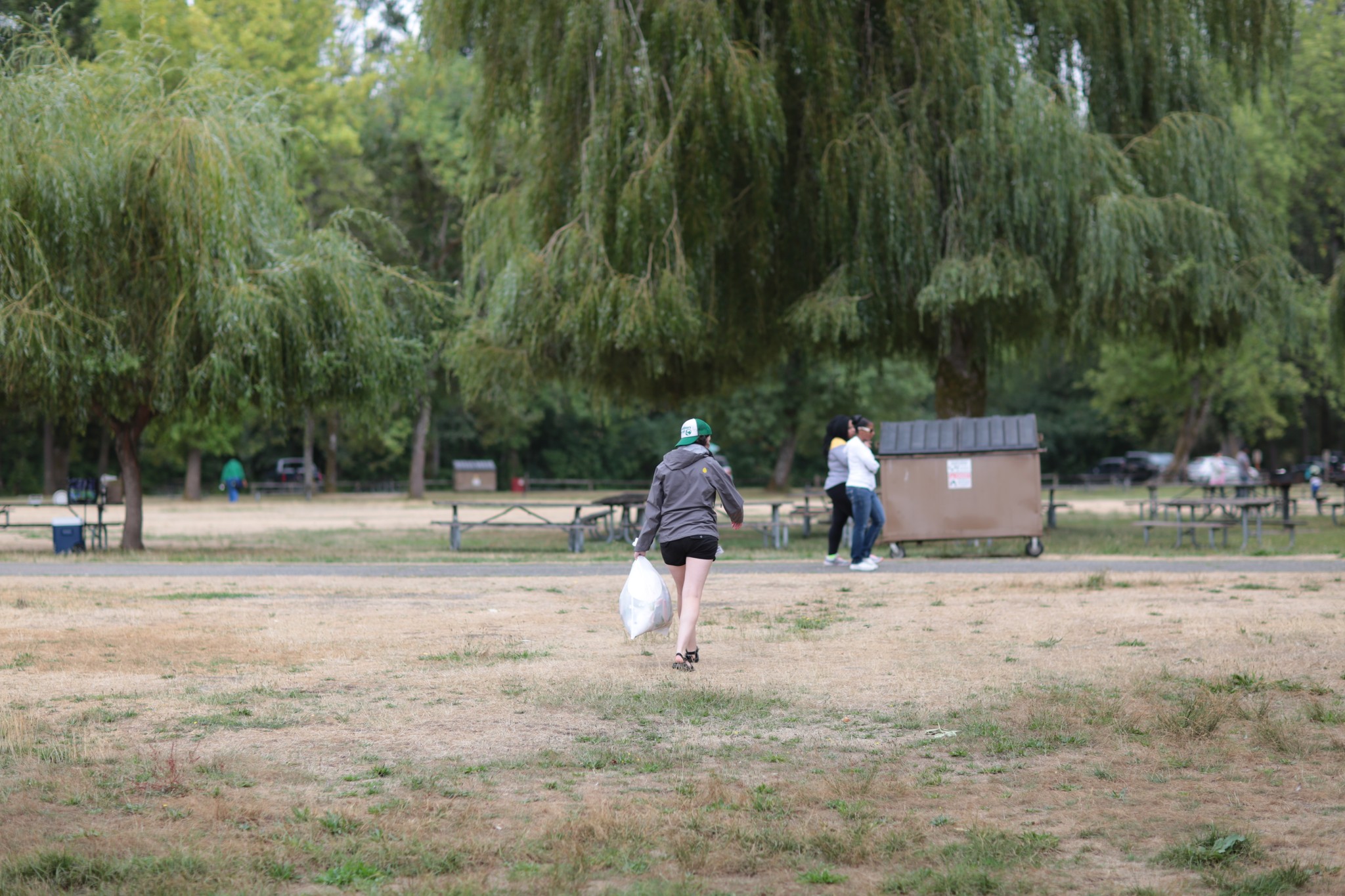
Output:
[51,517,85,552]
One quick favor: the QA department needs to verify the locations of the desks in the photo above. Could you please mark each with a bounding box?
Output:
[0,500,108,551]
[250,482,313,504]
[787,492,831,539]
[593,492,648,544]
[1143,480,1277,552]
[431,499,588,553]
[1041,483,1078,528]
[714,499,793,549]
[1124,498,1288,504]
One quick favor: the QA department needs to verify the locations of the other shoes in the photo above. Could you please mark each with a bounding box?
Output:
[869,554,883,563]
[850,558,878,571]
[824,554,852,567]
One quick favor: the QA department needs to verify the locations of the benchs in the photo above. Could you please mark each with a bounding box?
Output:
[570,509,617,523]
[790,506,833,515]
[1210,520,1312,553]
[432,521,596,530]
[1042,504,1067,508]
[719,521,789,527]
[1132,519,1231,549]
[0,523,122,526]
[1321,502,1345,526]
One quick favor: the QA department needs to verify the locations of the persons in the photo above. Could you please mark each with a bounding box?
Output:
[822,413,885,571]
[1102,448,1324,515]
[219,455,247,502]
[708,442,733,555]
[633,417,743,672]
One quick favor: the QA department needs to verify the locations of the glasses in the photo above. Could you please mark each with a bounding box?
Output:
[857,426,874,432]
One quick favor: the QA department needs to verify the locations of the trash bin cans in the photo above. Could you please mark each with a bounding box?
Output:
[878,411,1048,542]
[451,459,498,491]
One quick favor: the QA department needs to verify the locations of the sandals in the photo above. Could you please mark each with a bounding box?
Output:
[687,645,700,662]
[672,653,694,672]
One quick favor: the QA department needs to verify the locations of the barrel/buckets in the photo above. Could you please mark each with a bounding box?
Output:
[51,517,84,554]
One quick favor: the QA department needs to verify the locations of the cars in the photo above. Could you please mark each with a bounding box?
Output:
[1090,447,1345,490]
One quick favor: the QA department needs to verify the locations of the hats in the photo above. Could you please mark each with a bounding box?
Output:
[675,417,712,447]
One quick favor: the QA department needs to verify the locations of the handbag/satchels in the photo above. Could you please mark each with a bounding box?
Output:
[618,554,674,639]
